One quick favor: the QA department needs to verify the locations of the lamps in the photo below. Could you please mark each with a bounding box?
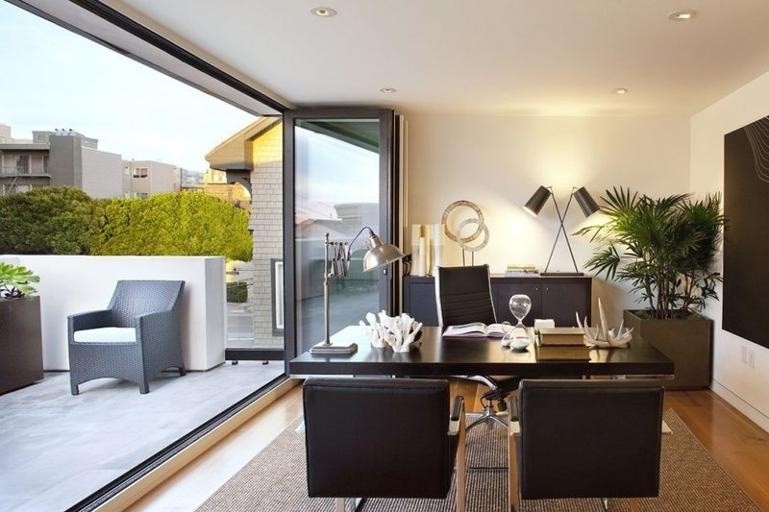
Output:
[668,9,696,23]
[309,7,338,17]
[310,225,407,357]
[379,87,396,94]
[522,185,601,277]
[612,88,628,97]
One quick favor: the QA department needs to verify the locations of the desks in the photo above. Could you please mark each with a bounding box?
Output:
[288,323,674,376]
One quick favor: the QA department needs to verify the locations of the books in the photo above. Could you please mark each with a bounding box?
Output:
[442,321,516,338]
[535,337,593,361]
[538,327,586,346]
[507,264,540,277]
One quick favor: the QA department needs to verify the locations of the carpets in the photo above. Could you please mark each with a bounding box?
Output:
[192,406,765,511]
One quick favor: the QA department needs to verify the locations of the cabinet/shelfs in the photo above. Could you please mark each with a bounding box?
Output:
[401,272,592,327]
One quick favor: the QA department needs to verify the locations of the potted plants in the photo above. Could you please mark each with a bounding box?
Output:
[567,185,730,391]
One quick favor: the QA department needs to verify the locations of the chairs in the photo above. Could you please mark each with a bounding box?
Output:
[507,377,664,512]
[301,377,468,512]
[66,279,186,396]
[433,264,515,437]
